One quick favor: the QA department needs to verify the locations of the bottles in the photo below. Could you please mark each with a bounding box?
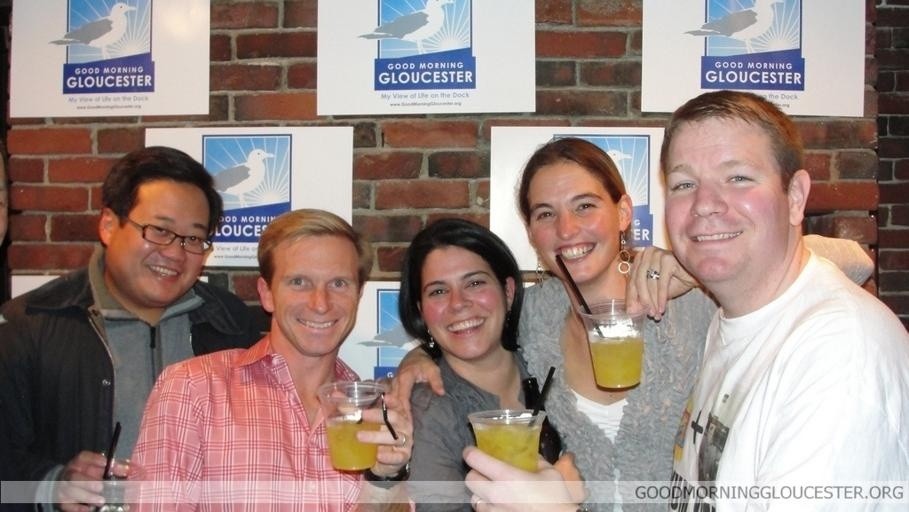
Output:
[518,375,565,469]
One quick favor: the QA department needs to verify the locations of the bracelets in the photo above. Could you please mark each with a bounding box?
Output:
[402,433,406,447]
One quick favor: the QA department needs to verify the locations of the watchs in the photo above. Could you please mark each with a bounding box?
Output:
[364,462,410,490]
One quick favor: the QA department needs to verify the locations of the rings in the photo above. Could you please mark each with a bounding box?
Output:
[647,269,660,279]
[475,498,482,511]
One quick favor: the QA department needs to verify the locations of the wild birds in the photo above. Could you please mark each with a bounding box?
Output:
[50,2,136,61]
[358,325,423,352]
[359,0,456,56]
[208,148,276,209]
[606,149,632,167]
[683,1,785,55]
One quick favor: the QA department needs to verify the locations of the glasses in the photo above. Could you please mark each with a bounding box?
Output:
[120,215,211,254]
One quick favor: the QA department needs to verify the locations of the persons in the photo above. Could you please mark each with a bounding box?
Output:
[398,217,590,512]
[660,89,909,512]
[0,146,263,512]
[123,209,414,512]
[384,140,875,512]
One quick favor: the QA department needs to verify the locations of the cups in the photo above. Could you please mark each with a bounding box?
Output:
[466,407,547,472]
[317,379,391,473]
[576,298,650,391]
[93,461,128,512]
